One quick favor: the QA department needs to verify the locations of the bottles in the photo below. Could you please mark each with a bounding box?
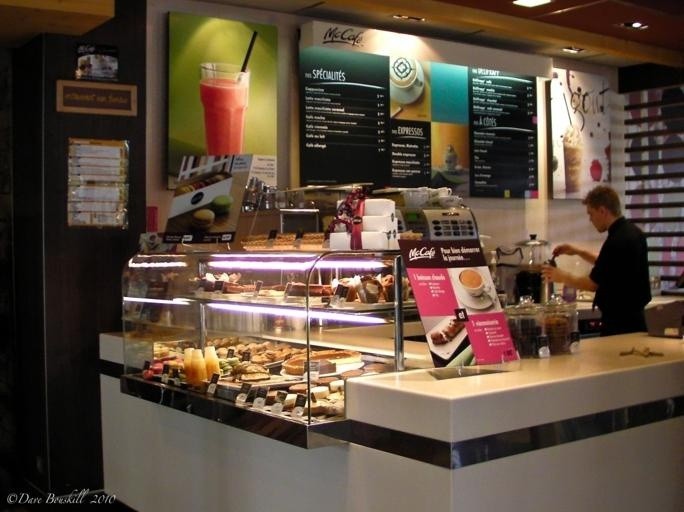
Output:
[245,176,257,211]
[204,346,220,380]
[192,349,207,386]
[504,295,545,357]
[260,185,272,209]
[184,348,194,384]
[545,293,579,354]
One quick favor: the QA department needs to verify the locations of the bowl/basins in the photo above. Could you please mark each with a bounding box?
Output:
[390,54,423,89]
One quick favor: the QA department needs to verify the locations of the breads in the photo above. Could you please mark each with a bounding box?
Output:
[189,272,410,304]
[431,319,466,344]
[127,335,387,417]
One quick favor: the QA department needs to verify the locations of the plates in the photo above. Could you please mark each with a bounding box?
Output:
[390,59,425,104]
[280,362,365,378]
[426,315,468,360]
[241,290,283,299]
[444,166,462,172]
[358,300,416,308]
[456,282,495,310]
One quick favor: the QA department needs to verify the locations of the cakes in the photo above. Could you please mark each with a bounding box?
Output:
[192,209,214,230]
[213,195,233,215]
[175,175,226,197]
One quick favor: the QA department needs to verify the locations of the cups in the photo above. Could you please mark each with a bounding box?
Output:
[458,269,490,296]
[275,190,287,208]
[200,63,250,155]
[569,327,570,329]
[445,156,460,169]
[564,126,582,191]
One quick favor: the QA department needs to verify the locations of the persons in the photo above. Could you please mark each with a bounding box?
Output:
[540,184,653,336]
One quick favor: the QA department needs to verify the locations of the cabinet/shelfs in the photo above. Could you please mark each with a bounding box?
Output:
[121,253,401,450]
